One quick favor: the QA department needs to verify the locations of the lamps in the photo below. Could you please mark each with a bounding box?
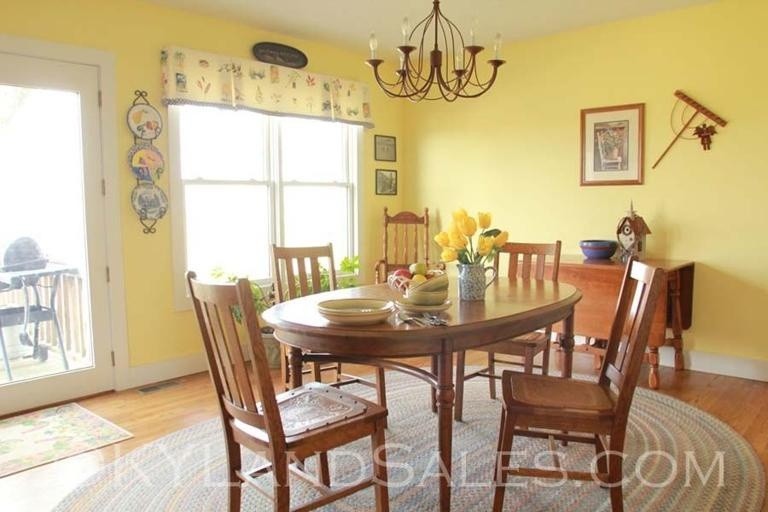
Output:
[364,0,506,106]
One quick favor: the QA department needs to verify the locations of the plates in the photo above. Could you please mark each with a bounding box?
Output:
[318,298,397,324]
[251,41,308,69]
[127,103,168,220]
[395,275,453,315]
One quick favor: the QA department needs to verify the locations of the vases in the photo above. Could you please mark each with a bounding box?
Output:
[452,260,500,303]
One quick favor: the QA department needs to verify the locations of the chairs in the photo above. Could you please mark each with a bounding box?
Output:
[453,236,564,425]
[0,262,71,382]
[375,202,440,283]
[267,239,390,430]
[180,269,393,512]
[487,254,665,512]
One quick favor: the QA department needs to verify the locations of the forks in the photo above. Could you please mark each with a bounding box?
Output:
[397,311,447,327]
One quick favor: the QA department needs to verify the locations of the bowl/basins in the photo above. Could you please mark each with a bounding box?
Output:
[580,240,618,259]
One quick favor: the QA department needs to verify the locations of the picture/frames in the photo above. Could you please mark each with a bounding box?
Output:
[375,167,399,198]
[578,100,647,187]
[372,132,399,163]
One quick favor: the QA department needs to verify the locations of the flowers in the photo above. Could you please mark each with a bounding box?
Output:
[431,208,511,267]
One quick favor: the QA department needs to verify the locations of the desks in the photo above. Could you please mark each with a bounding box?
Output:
[499,251,694,391]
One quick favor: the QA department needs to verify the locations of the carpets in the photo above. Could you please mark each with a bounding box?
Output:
[47,360,764,512]
[0,400,135,483]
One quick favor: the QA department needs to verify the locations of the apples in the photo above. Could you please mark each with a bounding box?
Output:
[393,262,443,289]
